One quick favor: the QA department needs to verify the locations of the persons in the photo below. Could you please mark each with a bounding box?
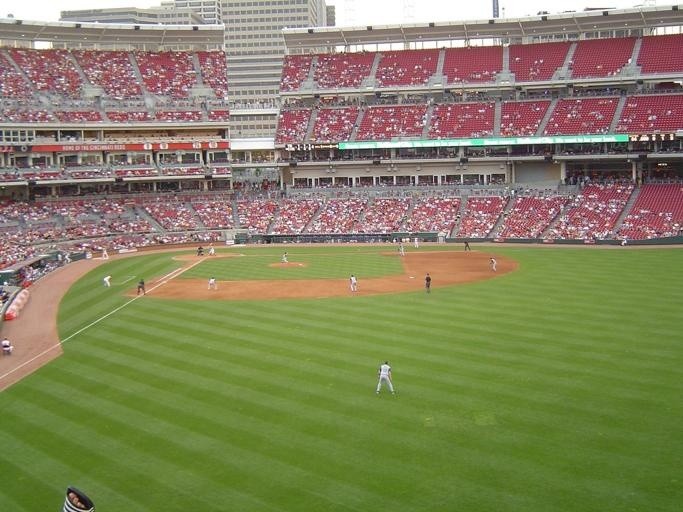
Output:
[376,361,395,396]
[2,337,14,356]
[2,49,683,305]
